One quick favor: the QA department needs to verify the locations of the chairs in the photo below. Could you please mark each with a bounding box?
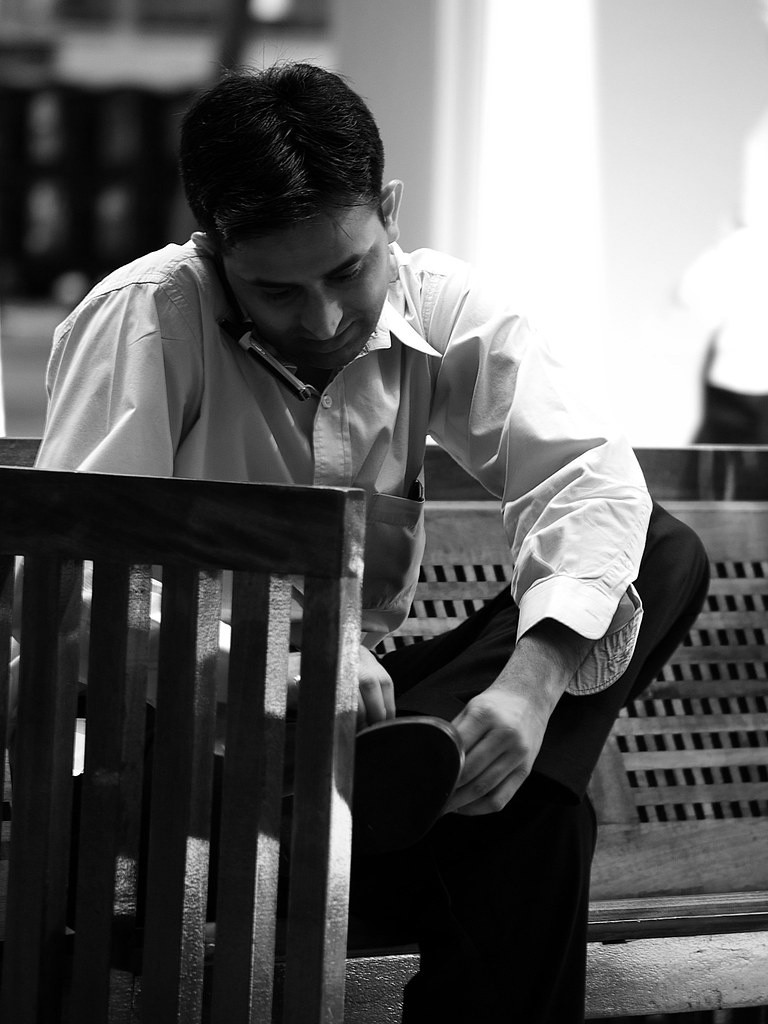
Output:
[0,467,367,1024]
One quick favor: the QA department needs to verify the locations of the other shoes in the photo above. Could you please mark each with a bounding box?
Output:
[281,716,465,864]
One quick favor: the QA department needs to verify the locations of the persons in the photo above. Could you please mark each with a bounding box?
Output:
[7,61,710,1024]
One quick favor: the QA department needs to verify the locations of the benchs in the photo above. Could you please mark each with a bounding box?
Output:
[0,438,768,945]
